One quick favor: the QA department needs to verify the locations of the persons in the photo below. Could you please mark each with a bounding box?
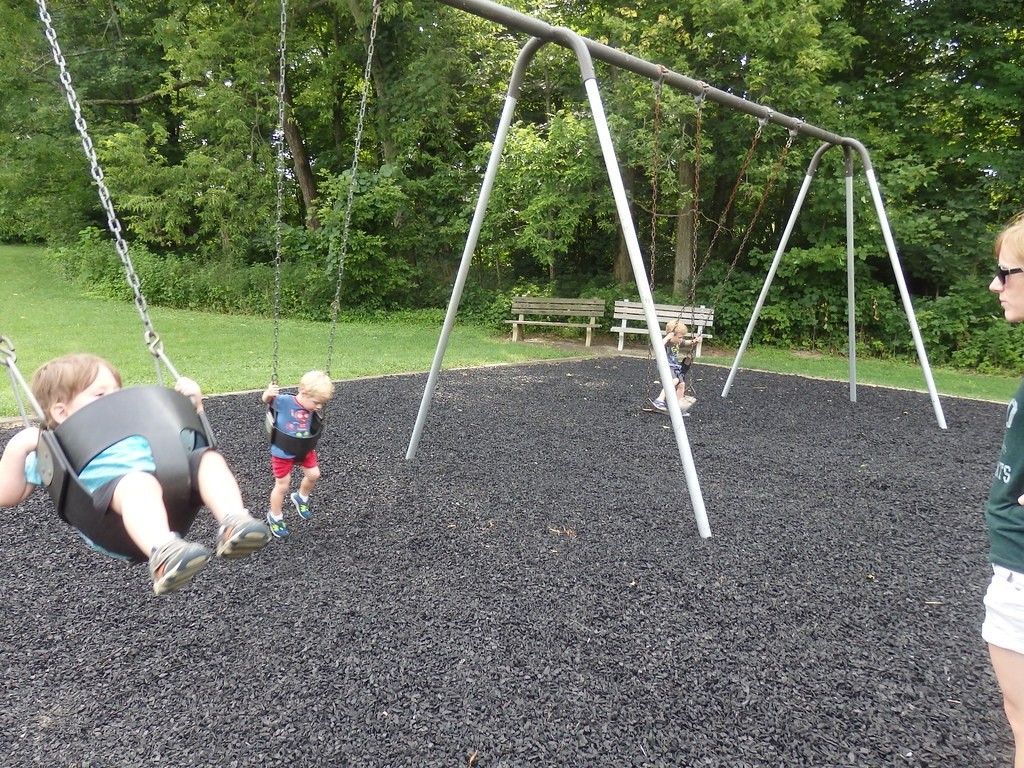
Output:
[652,320,703,418]
[261,369,336,540]
[980,219,1024,768]
[0,353,274,597]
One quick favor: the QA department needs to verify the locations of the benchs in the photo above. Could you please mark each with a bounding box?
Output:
[610,299,713,358]
[504,297,605,346]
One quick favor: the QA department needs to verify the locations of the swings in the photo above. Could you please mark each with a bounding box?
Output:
[1,0,219,558]
[637,78,800,416]
[262,0,382,466]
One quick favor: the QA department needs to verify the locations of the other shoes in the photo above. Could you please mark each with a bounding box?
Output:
[215,511,273,560]
[147,538,214,597]
[290,492,312,520]
[652,399,667,412]
[266,511,289,538]
[681,411,690,417]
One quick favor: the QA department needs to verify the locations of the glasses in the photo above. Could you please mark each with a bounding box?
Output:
[995,264,1023,285]
